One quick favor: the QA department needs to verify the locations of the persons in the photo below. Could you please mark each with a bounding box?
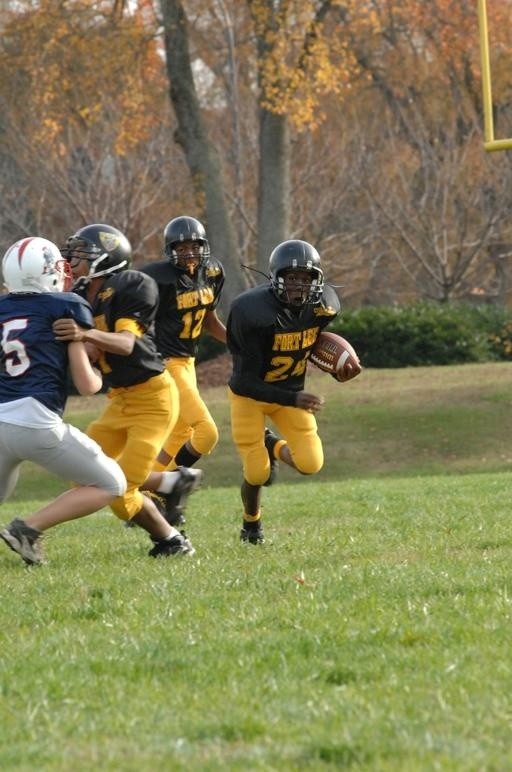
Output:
[0,221,204,558]
[125,213,229,532]
[0,236,130,570]
[224,238,363,547]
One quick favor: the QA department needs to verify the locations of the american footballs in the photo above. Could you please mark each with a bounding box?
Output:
[308,332,361,373]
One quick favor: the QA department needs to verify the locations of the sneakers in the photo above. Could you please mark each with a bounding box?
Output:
[120,462,203,530]
[263,425,280,487]
[1,515,50,568]
[144,529,196,560]
[239,527,266,548]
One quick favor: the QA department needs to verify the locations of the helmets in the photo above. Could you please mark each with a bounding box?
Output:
[57,222,134,291]
[266,237,325,308]
[163,213,212,270]
[1,235,66,296]
